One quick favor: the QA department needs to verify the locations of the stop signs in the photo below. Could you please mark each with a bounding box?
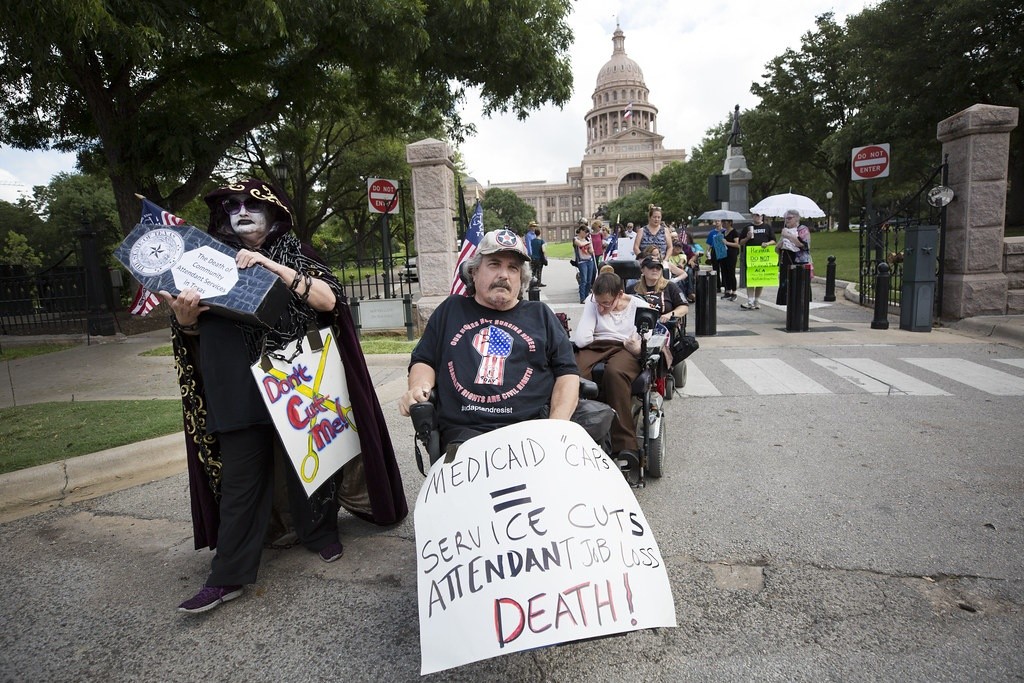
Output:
[850,143,892,180]
[367,178,400,214]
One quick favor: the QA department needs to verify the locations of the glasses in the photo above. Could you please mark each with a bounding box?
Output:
[598,226,601,228]
[221,197,268,215]
[651,207,661,209]
[785,217,793,220]
[628,226,632,228]
[589,291,618,309]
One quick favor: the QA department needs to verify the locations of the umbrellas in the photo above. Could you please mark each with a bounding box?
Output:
[698,210,746,221]
[750,186,827,228]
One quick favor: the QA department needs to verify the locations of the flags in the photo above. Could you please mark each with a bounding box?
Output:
[449,200,484,297]
[129,284,162,318]
[624,102,632,118]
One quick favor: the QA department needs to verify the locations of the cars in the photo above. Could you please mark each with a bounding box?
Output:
[401,240,462,282]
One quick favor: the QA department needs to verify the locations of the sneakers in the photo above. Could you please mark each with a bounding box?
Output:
[177,583,244,614]
[319,539,343,562]
[741,302,756,309]
[754,302,760,309]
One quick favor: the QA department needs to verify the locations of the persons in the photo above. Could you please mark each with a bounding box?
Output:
[399,229,580,452]
[525,221,548,287]
[159,177,408,615]
[727,104,741,146]
[573,205,812,473]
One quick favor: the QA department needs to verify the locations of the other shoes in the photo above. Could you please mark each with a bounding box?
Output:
[581,301,585,304]
[688,293,695,300]
[669,323,700,367]
[721,292,738,301]
[619,448,639,469]
[538,284,546,287]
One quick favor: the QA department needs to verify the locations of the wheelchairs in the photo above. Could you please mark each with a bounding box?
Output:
[656,302,692,388]
[554,314,668,489]
[406,390,445,476]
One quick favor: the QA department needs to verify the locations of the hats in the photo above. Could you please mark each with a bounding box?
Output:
[641,256,664,270]
[577,218,589,224]
[527,220,539,228]
[477,229,533,262]
[674,241,683,248]
[636,252,653,260]
[671,232,678,237]
